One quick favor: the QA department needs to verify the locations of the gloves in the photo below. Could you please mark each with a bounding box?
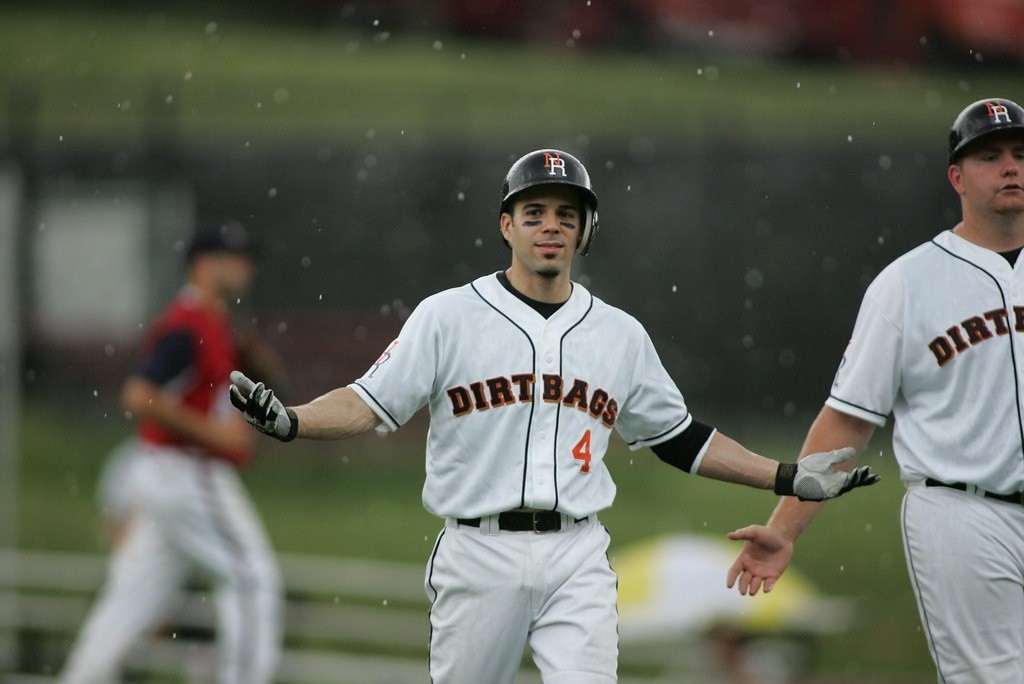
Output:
[774,447,881,502]
[229,371,298,443]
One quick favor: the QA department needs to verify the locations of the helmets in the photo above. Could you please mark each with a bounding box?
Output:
[185,215,264,260]
[948,98,1024,158]
[499,149,599,249]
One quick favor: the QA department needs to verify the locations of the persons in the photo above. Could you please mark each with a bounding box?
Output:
[224,146,881,684]
[53,214,283,684]
[726,98,1024,683]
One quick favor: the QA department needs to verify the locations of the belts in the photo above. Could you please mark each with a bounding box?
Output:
[926,478,1024,505]
[457,506,589,534]
